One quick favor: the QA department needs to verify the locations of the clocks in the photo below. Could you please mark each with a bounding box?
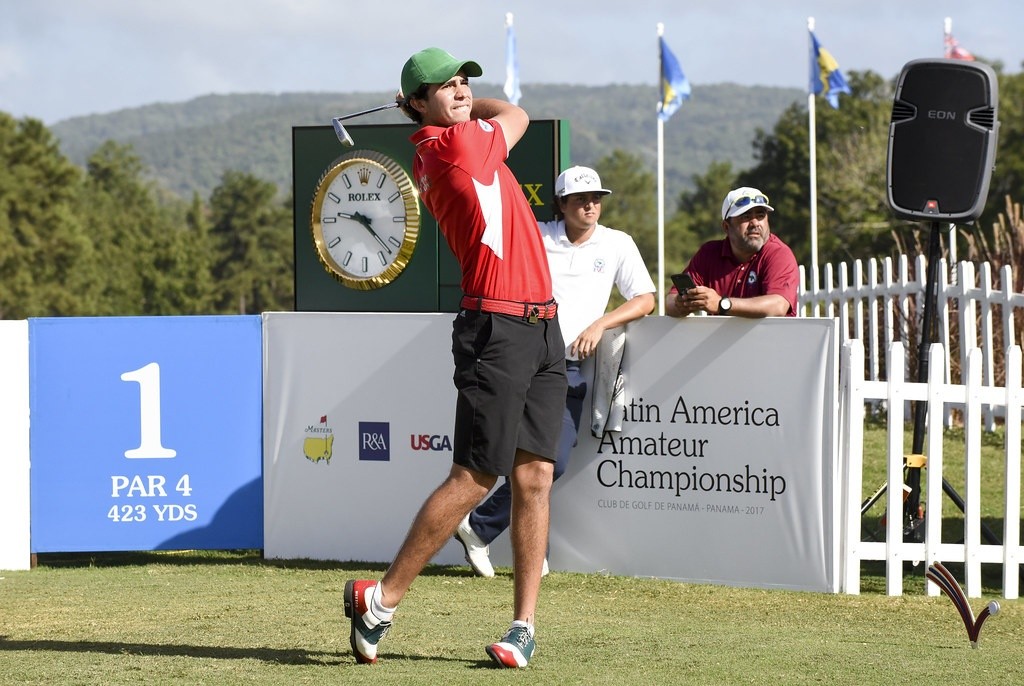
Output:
[308,150,421,291]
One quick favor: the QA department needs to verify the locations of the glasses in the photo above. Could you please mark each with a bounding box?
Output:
[725,194,769,220]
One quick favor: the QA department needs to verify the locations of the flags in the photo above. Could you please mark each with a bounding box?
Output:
[807,28,850,111]
[655,37,694,122]
[944,36,976,64]
[503,25,523,109]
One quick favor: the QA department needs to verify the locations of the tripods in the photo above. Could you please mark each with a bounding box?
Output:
[861,220,1004,573]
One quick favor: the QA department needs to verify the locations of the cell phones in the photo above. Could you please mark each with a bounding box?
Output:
[672,274,697,296]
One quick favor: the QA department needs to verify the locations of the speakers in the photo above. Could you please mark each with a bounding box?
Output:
[885,58,1001,225]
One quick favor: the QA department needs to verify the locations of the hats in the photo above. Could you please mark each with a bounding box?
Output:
[401,48,482,100]
[555,166,612,196]
[722,187,774,220]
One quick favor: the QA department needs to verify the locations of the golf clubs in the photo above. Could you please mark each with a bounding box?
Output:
[330,99,409,148]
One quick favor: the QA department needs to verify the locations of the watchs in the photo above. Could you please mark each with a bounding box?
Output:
[719,294,732,315]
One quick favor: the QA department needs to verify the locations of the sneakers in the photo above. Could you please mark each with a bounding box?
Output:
[485,627,535,668]
[540,558,549,576]
[343,579,398,664]
[453,511,494,578]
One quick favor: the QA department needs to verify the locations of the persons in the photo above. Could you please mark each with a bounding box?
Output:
[340,46,567,668]
[664,186,799,321]
[447,163,658,579]
